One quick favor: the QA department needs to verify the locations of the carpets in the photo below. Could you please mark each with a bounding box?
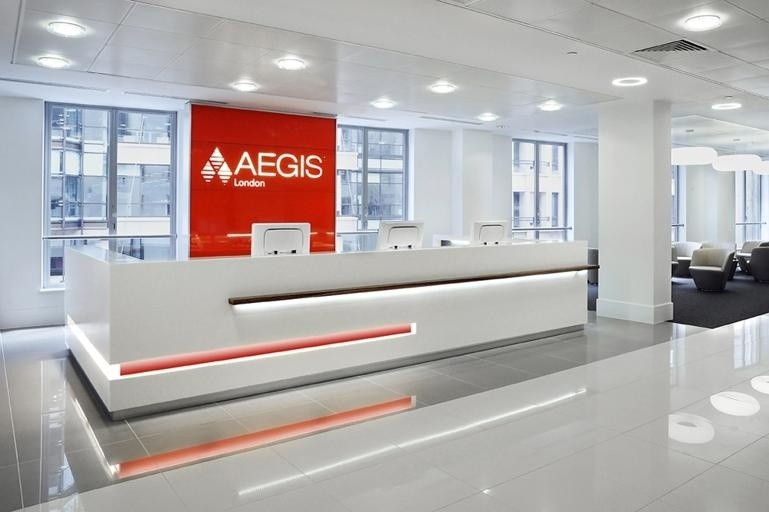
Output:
[588,271,769,329]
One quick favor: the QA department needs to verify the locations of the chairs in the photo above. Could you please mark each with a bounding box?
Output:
[672,241,769,292]
[588,248,598,285]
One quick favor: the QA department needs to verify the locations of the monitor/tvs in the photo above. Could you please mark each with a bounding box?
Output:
[376,219,424,250]
[473,220,508,244]
[251,222,310,255]
